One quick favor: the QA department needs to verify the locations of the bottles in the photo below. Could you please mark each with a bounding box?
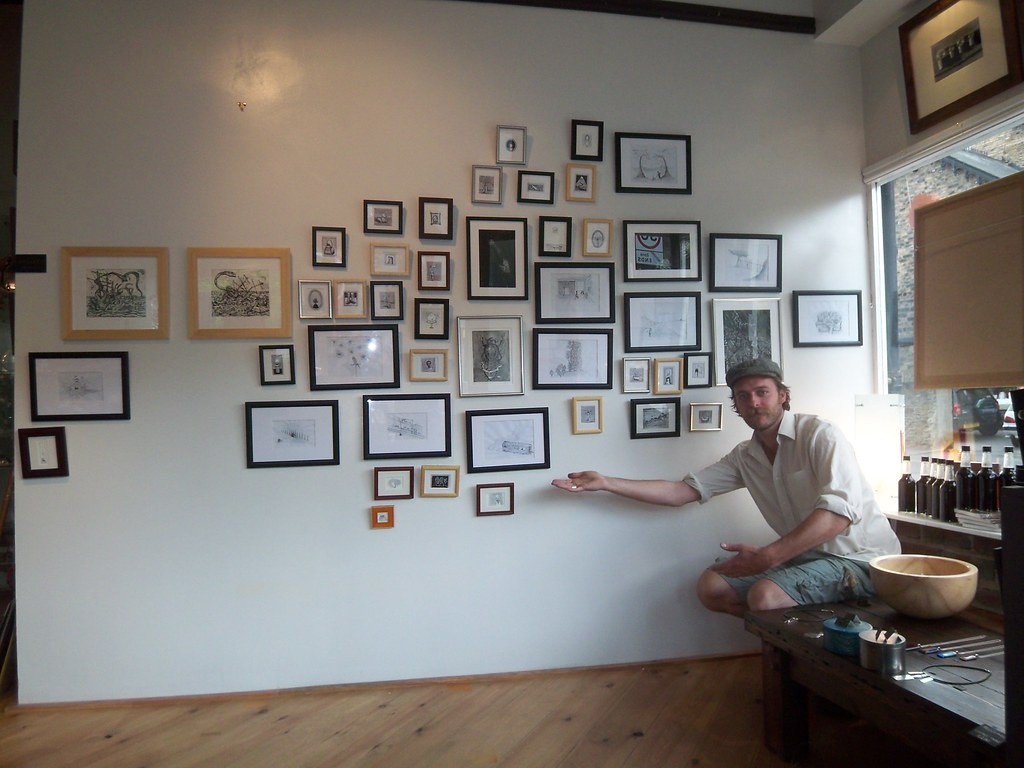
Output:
[940,460,958,523]
[932,459,945,520]
[1000,447,1018,510]
[926,458,938,517]
[898,456,915,515]
[917,457,930,515]
[955,446,977,511]
[976,446,998,513]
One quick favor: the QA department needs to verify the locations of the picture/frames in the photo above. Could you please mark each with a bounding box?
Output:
[467,214,532,303]
[898,0,1024,136]
[621,357,651,393]
[465,407,550,474]
[476,482,515,517]
[495,124,528,165]
[653,358,683,394]
[622,219,702,282]
[533,261,617,325]
[706,230,863,387]
[570,396,603,435]
[581,217,614,259]
[689,402,724,431]
[624,291,702,353]
[566,163,597,202]
[470,164,503,205]
[456,314,526,397]
[538,214,572,257]
[13,192,460,529]
[629,397,681,440]
[570,119,604,162]
[684,352,712,390]
[515,170,557,206]
[615,131,694,197]
[533,328,613,390]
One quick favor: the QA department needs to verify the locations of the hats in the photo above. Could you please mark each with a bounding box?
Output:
[726,359,782,387]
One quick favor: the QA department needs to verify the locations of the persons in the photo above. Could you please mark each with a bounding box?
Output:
[550,358,902,619]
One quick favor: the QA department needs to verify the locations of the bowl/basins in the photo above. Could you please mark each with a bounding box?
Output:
[869,554,978,620]
[859,630,906,674]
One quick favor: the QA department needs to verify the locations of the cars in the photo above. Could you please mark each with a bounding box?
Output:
[952,387,1021,447]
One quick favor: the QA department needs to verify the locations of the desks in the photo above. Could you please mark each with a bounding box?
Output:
[747,592,1007,768]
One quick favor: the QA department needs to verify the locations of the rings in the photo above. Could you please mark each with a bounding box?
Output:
[572,484,577,488]
